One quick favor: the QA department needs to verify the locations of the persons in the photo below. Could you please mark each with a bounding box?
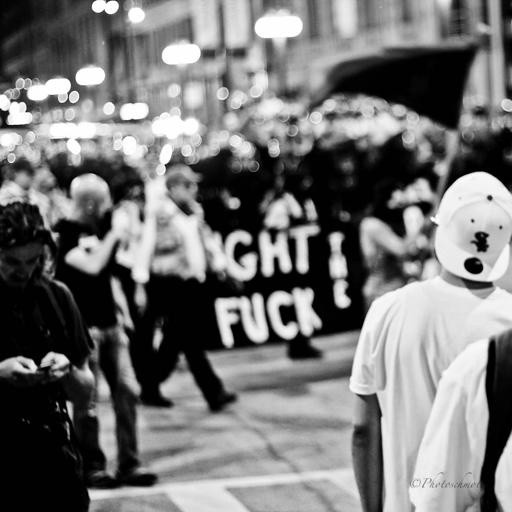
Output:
[1,102,510,512]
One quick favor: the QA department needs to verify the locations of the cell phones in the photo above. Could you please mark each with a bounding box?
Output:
[36,365,51,373]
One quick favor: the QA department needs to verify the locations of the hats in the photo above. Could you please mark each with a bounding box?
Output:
[164,163,203,189]
[431,171,512,281]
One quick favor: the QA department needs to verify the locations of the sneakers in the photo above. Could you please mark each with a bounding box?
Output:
[286,343,324,361]
[137,389,176,409]
[208,390,240,414]
[85,464,160,493]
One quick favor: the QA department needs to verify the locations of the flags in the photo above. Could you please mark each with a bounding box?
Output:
[300,35,483,132]
